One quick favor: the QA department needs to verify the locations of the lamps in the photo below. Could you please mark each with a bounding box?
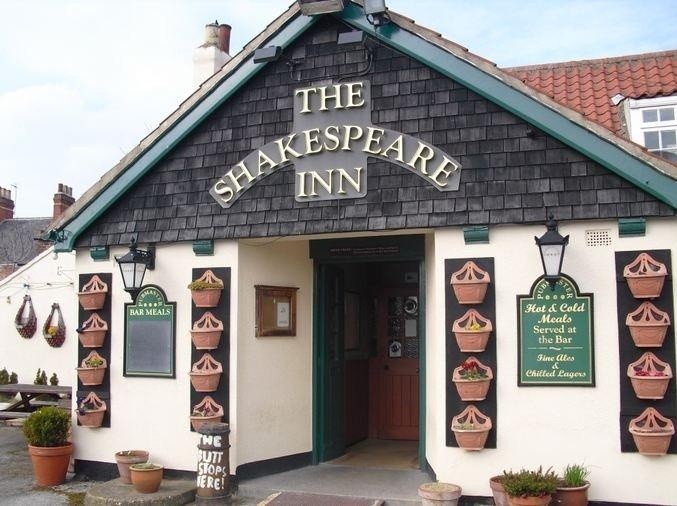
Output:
[534,213,570,292]
[113,235,155,305]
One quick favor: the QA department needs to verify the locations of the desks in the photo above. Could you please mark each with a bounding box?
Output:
[0,383,71,412]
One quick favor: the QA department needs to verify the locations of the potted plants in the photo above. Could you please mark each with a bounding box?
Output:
[22,407,74,486]
[115,449,165,492]
[488,461,592,506]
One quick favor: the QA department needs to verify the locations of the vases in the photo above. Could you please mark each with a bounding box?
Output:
[450,258,491,452]
[75,274,109,430]
[417,482,462,506]
[14,294,67,348]
[188,269,224,435]
[621,252,675,456]
